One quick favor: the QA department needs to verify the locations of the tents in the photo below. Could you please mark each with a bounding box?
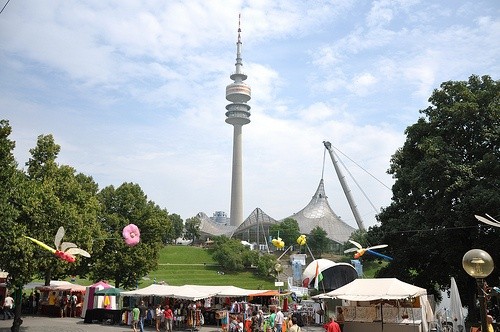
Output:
[311,278,434,332]
[32,278,282,300]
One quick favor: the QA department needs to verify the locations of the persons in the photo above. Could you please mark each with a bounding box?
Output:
[22,291,78,319]
[327,307,345,332]
[0,294,16,320]
[132,300,284,332]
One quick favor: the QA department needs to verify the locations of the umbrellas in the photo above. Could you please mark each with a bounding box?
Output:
[450,277,466,332]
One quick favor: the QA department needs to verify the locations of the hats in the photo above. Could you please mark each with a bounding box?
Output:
[277,307,281,310]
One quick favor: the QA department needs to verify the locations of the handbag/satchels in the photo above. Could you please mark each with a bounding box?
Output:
[10,304,12,309]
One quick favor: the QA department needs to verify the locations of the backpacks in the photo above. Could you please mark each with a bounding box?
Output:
[71,296,75,305]
